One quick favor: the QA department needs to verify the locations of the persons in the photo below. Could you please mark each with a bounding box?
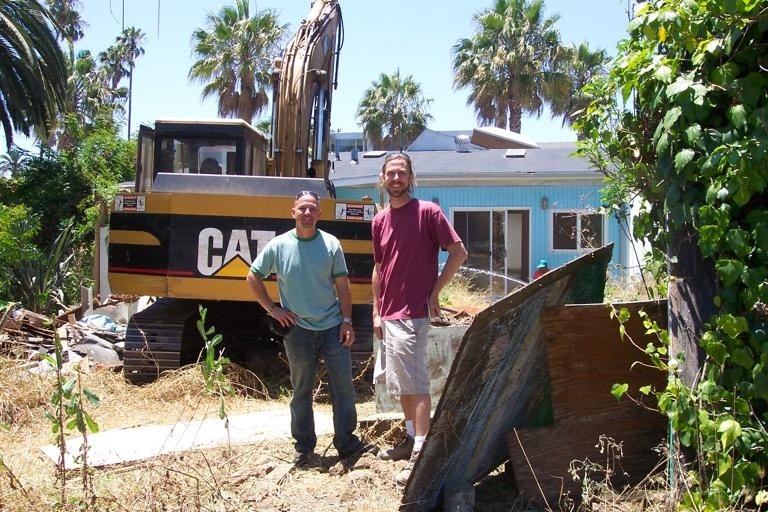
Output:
[199,157,222,174]
[533,259,552,283]
[371,152,469,462]
[245,191,362,468]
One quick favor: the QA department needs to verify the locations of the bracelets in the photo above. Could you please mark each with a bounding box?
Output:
[266,305,276,318]
[340,317,353,326]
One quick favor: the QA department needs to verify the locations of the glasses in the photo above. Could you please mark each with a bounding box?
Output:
[297,191,319,200]
[385,153,409,162]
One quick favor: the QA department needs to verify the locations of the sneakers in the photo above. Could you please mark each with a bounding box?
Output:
[338,443,376,458]
[381,435,420,461]
[294,451,312,463]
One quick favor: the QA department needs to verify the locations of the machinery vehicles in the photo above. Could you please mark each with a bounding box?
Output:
[102,1,387,397]
